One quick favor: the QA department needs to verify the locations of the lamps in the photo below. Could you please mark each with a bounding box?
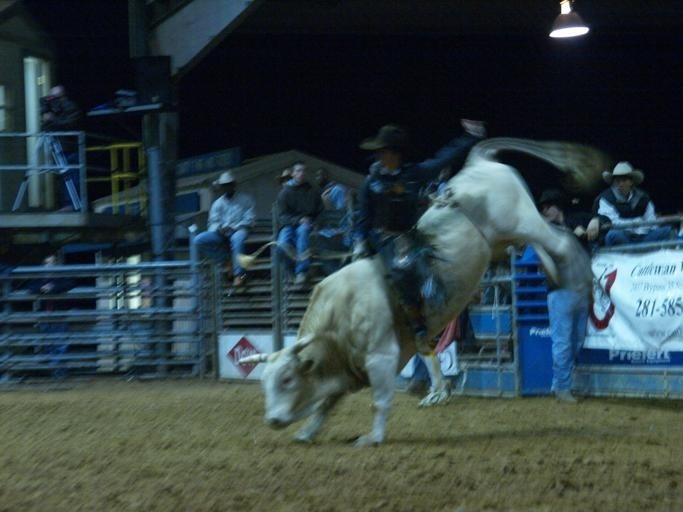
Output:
[547,0,592,40]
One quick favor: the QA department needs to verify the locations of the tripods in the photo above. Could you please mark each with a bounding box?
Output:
[11,132,83,213]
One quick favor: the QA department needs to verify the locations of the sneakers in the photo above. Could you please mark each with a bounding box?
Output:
[293,271,310,287]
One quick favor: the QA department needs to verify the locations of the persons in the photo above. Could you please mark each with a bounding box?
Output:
[30,254,77,381]
[390,309,477,396]
[211,167,449,272]
[352,118,487,357]
[89,88,162,187]
[276,160,325,292]
[182,170,256,295]
[592,160,672,251]
[537,187,612,404]
[112,306,164,347]
[0,262,25,384]
[37,85,86,212]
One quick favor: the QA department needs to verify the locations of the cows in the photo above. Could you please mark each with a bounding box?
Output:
[236,137,616,448]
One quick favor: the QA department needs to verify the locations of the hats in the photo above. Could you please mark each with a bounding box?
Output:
[275,168,293,179]
[601,161,644,185]
[217,172,235,185]
[358,125,414,152]
[535,191,569,204]
